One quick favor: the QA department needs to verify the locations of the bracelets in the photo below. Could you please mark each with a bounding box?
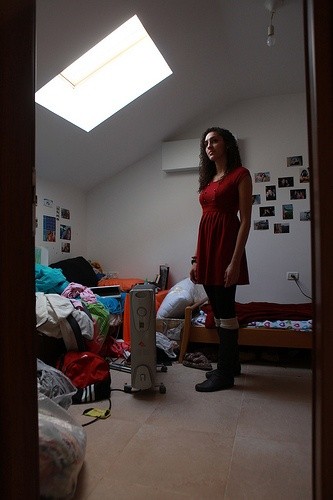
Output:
[191,257,196,264]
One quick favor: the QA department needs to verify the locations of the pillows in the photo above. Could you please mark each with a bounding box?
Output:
[156,276,195,320]
[97,278,145,291]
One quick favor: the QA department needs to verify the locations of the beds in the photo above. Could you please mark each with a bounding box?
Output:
[179,296,312,365]
[35,266,170,353]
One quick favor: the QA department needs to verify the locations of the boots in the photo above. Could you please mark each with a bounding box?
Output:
[195,329,239,391]
[206,327,242,377]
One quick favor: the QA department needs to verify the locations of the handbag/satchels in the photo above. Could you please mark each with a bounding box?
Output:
[57,349,111,405]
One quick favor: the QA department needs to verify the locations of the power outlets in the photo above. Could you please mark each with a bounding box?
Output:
[286,272,298,279]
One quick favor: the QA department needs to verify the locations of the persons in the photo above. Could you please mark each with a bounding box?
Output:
[189,127,253,391]
[253,158,310,232]
[60,209,71,253]
